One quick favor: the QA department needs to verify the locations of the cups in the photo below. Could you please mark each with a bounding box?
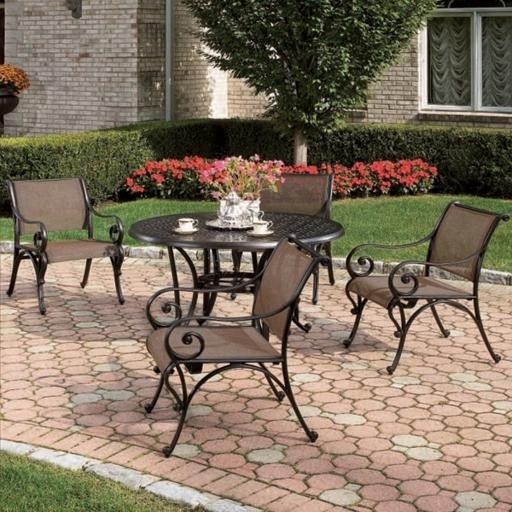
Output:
[177,216,199,230]
[253,220,275,232]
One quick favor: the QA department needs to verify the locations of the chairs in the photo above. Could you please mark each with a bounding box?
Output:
[6,176,125,314]
[143,234,329,457]
[229,172,336,304]
[343,199,510,373]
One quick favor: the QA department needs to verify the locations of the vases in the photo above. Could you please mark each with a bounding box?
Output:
[217,195,251,228]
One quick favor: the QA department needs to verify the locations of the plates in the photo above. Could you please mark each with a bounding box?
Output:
[245,229,276,238]
[173,227,199,236]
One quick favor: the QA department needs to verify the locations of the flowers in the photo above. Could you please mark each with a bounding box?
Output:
[201,154,283,198]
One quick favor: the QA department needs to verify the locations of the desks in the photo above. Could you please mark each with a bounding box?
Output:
[129,211,344,332]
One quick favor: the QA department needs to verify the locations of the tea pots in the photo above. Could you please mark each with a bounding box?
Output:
[216,191,262,227]
